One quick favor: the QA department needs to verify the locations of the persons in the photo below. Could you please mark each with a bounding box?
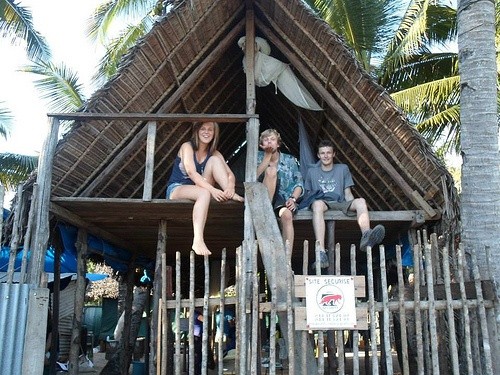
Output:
[190,295,235,373]
[297,140,386,269]
[256,129,305,267]
[165,121,245,256]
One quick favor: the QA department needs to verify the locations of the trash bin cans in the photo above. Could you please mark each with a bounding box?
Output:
[133,362,146,375]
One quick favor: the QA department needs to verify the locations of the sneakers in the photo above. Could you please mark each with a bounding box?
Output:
[360,225,385,252]
[312,253,329,268]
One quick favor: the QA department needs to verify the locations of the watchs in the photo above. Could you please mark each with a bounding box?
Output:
[289,196,296,203]
